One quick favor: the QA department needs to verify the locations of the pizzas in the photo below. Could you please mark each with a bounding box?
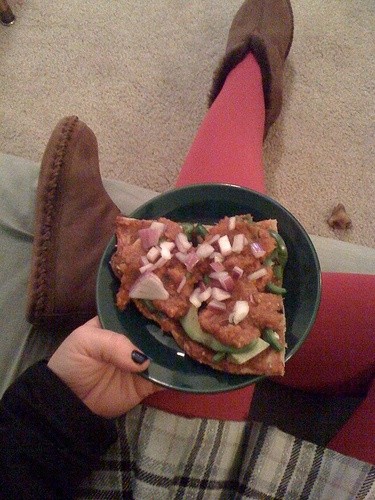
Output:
[110,213,286,377]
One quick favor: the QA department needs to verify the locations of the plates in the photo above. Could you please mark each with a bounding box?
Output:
[94,181,322,394]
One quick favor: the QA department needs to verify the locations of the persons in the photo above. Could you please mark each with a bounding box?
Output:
[0,0,375,500]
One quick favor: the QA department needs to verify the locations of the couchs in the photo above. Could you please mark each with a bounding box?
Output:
[0,152,375,401]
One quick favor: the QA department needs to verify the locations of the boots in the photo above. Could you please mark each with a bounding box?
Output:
[24,115,128,333]
[206,0,294,143]
